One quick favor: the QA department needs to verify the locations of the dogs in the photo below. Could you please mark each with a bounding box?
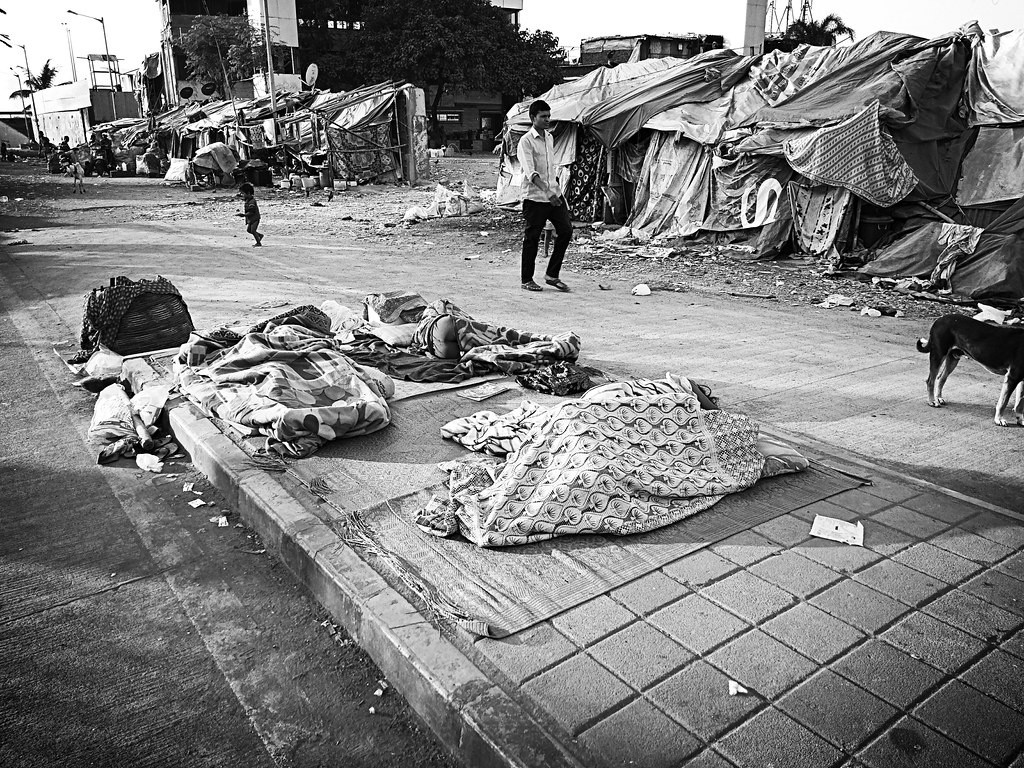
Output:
[916,314,1024,426]
[69,162,86,194]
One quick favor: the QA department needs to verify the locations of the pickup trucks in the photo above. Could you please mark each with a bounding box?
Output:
[5,143,43,163]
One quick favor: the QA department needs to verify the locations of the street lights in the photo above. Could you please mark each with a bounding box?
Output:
[67,10,117,121]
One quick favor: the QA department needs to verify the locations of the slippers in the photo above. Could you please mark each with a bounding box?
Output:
[547,278,570,292]
[522,281,543,291]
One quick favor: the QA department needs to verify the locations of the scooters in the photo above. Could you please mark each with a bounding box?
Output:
[47,139,114,177]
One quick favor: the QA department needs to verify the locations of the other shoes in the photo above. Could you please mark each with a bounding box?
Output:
[260,234,263,241]
[252,242,262,246]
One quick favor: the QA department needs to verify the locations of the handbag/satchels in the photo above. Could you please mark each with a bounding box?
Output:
[80,276,197,355]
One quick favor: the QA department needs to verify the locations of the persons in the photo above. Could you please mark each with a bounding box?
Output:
[238,183,264,247]
[38,130,116,178]
[517,100,572,292]
[1,143,6,161]
